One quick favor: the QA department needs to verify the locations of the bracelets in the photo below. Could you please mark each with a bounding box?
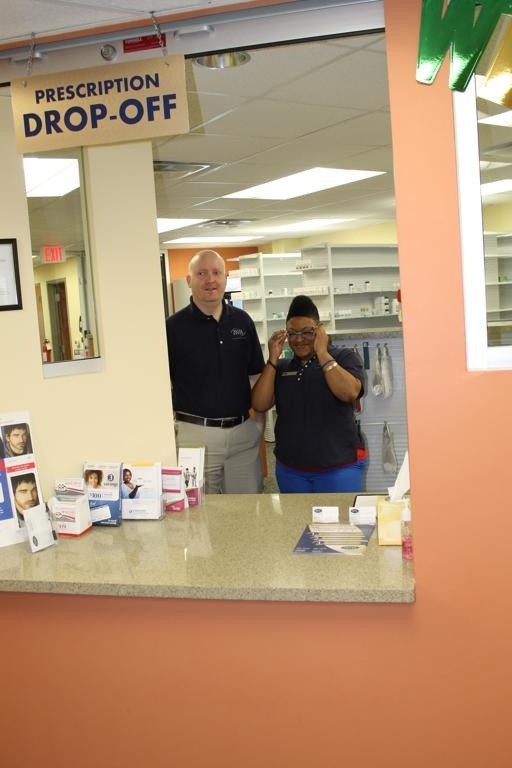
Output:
[319,360,339,373]
[268,359,279,372]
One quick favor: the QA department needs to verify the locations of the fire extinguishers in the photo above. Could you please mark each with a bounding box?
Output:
[42,339,51,363]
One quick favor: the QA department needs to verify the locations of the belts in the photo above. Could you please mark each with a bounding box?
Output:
[175,411,250,428]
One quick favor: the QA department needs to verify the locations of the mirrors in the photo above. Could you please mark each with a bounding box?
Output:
[20,146,106,374]
[152,27,410,496]
[454,4,512,372]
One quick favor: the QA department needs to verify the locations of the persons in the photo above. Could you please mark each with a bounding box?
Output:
[121,471,143,500]
[251,294,368,494]
[166,248,265,493]
[3,423,33,457]
[85,471,103,489]
[10,473,40,527]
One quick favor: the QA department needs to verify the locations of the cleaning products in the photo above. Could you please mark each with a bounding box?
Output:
[396,499,412,559]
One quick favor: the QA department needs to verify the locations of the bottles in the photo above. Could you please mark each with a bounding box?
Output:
[349,282,354,291]
[273,312,287,318]
[399,498,413,559]
[284,288,290,295]
[363,282,370,292]
[392,298,400,313]
[361,308,369,315]
[83,330,95,359]
[269,290,273,298]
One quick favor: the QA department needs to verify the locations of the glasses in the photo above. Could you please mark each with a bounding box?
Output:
[286,330,314,339]
